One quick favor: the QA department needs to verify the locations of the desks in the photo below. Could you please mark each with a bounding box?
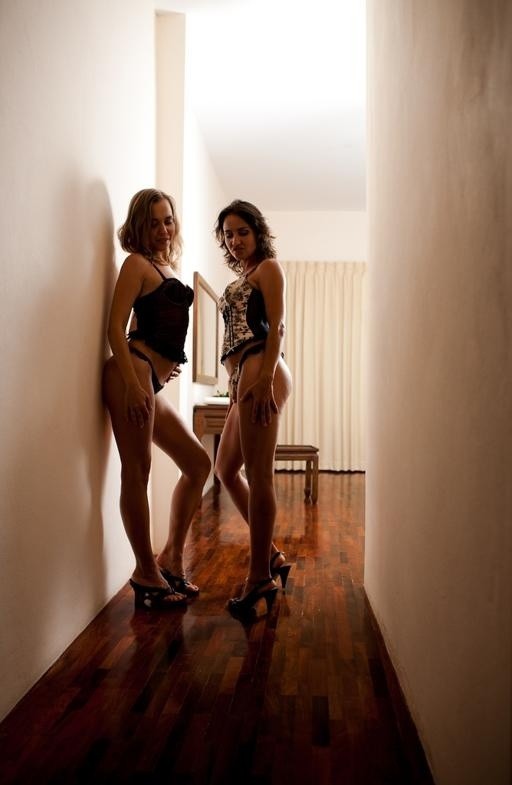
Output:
[190,406,228,510]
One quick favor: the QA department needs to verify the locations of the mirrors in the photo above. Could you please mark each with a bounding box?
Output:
[190,272,221,384]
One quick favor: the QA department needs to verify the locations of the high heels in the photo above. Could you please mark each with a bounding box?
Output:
[224,551,292,612]
[129,567,200,605]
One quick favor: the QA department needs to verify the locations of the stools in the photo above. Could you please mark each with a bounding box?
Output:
[274,444,318,504]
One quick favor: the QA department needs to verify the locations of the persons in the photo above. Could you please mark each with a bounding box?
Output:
[212,197,294,617]
[101,186,214,610]
